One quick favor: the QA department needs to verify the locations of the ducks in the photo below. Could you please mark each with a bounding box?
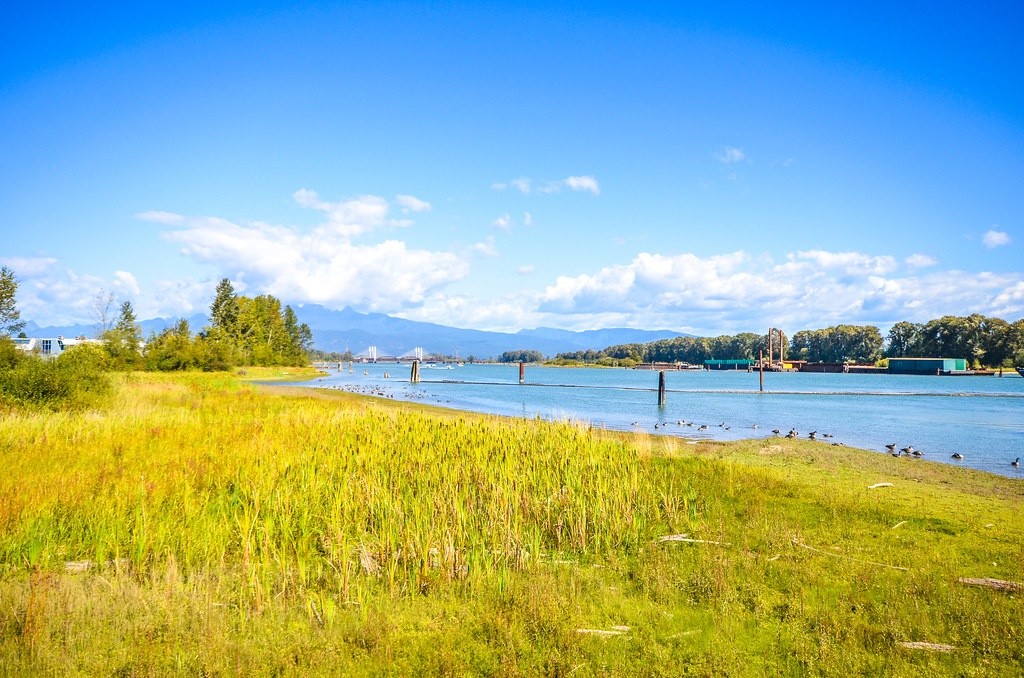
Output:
[630,422,638,425]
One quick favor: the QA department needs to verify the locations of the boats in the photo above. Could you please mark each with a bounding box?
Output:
[1016,366,1024,377]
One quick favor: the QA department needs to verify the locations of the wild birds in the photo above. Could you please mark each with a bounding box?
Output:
[719,422,731,430]
[328,384,452,402]
[808,431,817,440]
[831,442,843,446]
[785,428,799,438]
[822,433,833,438]
[885,442,924,457]
[697,425,707,431]
[772,429,780,435]
[1011,457,1019,466]
[678,419,693,426]
[663,422,667,426]
[951,453,963,459]
[655,423,658,429]
[752,425,759,429]
